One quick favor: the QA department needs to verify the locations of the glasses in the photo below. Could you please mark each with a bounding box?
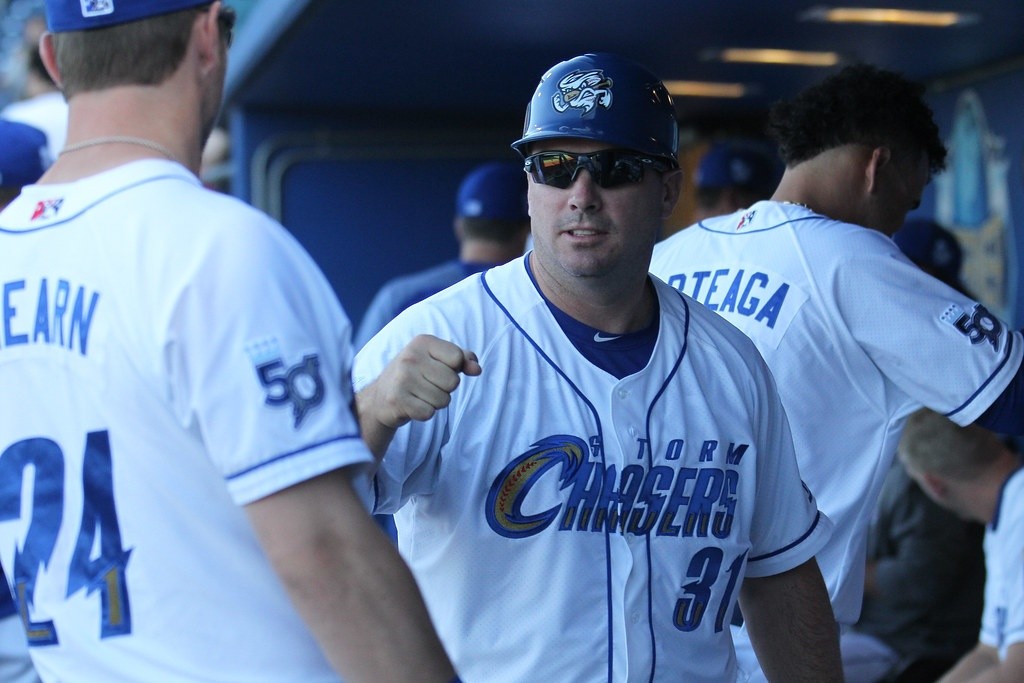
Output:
[524,149,670,189]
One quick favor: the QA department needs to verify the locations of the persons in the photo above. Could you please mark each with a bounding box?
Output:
[691,141,782,224]
[352,163,532,358]
[0,8,69,208]
[1,0,462,683]
[350,50,848,683]
[838,402,1023,683]
[647,58,1023,683]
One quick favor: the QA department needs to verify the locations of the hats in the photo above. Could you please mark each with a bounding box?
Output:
[457,165,528,216]
[894,219,977,302]
[700,140,778,190]
[43,0,209,33]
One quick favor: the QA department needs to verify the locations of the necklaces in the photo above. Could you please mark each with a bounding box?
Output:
[58,136,175,159]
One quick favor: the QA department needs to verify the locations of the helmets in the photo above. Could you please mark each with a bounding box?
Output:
[509,52,681,169]
